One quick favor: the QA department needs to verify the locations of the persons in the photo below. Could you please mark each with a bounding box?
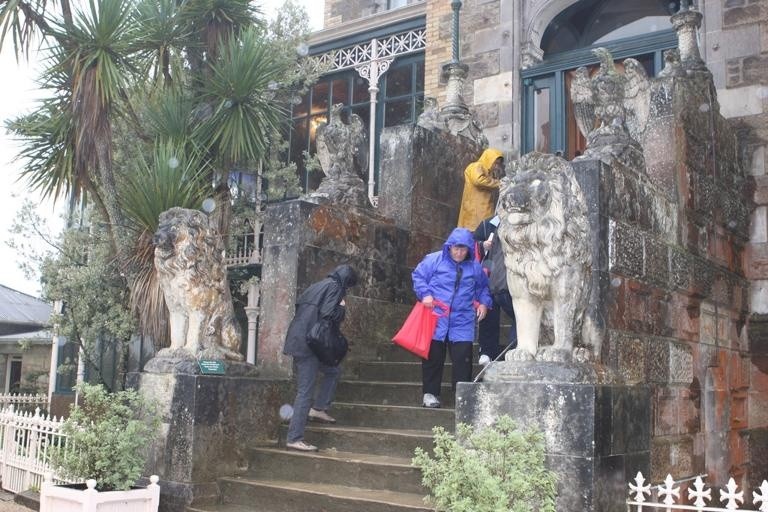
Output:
[455,148,506,232]
[409,227,495,409]
[282,264,358,451]
[471,208,518,365]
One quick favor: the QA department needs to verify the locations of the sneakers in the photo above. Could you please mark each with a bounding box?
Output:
[286,441,318,451]
[479,355,492,365]
[308,408,336,424]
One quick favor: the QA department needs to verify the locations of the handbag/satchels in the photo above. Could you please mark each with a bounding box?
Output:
[392,300,439,360]
[306,319,347,366]
[423,393,441,407]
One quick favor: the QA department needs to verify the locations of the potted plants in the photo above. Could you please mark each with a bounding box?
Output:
[36,378,164,512]
